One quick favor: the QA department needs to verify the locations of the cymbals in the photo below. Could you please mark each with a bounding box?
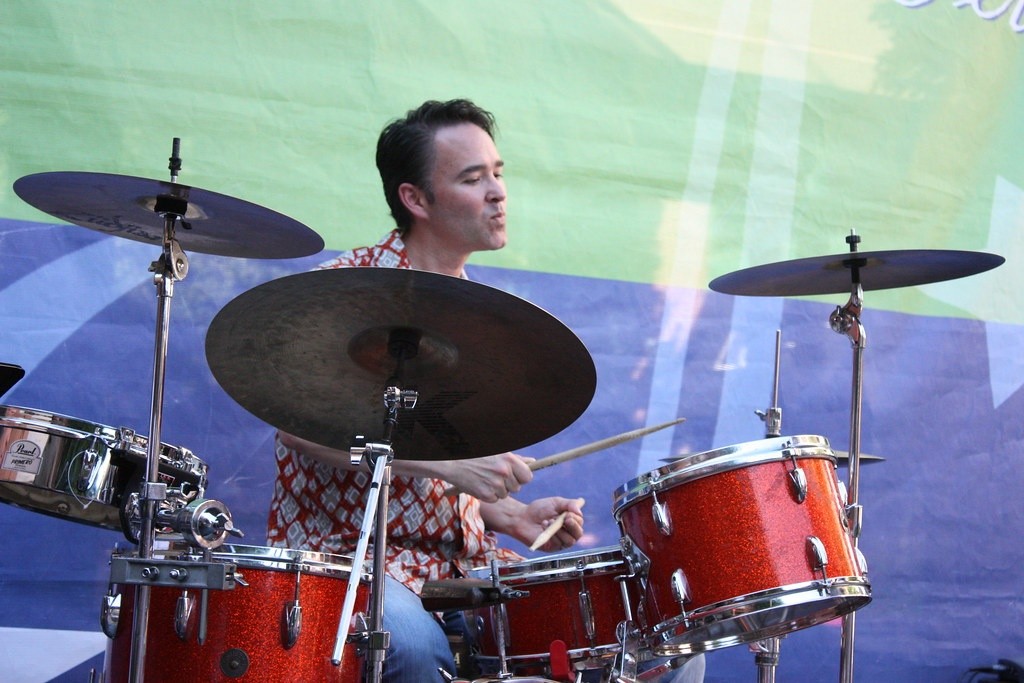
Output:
[709,231,1006,300]
[12,171,325,258]
[204,266,597,462]
[661,438,888,464]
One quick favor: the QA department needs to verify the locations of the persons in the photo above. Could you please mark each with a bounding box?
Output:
[264,97,584,683]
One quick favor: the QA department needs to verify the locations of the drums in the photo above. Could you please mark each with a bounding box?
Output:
[0,402,208,534]
[610,435,871,655]
[468,542,685,682]
[100,546,374,683]
